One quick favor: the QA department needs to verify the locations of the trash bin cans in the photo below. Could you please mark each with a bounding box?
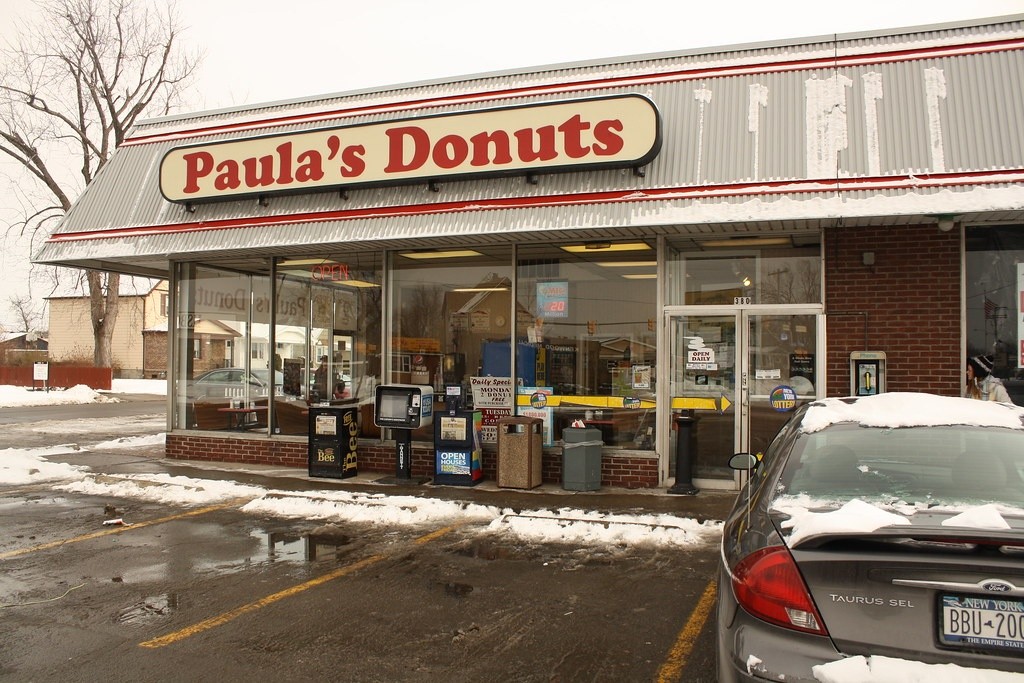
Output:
[561,422,603,490]
[499,416,542,489]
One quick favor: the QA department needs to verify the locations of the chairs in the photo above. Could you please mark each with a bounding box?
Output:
[806,445,895,493]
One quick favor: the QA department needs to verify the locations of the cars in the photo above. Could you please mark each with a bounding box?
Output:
[716,389,1024,682]
[176,367,284,413]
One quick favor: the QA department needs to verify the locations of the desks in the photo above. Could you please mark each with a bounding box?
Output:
[218,406,268,432]
[582,418,630,446]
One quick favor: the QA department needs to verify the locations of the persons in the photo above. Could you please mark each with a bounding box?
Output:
[312,355,350,399]
[965,355,1013,405]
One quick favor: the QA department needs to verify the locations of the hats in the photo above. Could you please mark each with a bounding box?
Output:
[967,354,995,379]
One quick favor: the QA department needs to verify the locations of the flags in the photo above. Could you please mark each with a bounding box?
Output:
[984,298,998,318]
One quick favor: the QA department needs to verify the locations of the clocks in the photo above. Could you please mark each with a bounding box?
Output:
[495,316,506,327]
